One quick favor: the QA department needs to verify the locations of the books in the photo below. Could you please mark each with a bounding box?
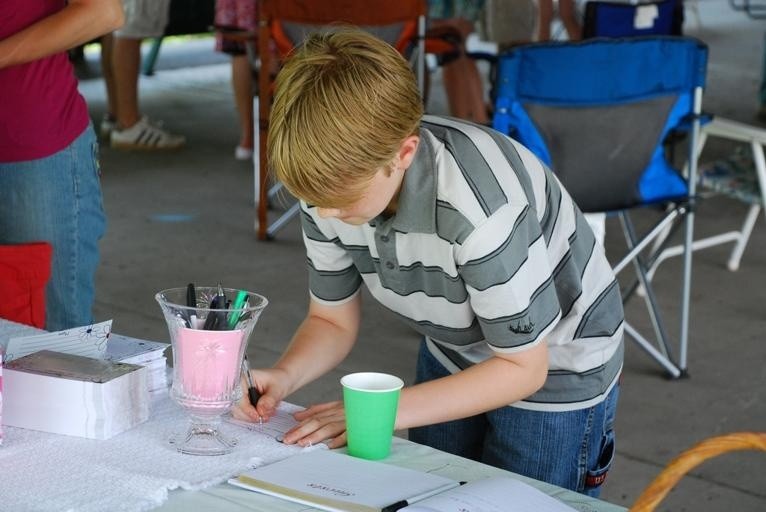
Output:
[225,445,456,512]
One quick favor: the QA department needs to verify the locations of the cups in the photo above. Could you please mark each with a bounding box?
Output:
[339,371,405,459]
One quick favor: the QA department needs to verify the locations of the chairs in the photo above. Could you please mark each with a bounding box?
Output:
[425,2,581,124]
[488,34,717,379]
[582,1,688,40]
[252,0,423,239]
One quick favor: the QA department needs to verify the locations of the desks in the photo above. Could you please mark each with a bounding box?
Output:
[0,317,631,512]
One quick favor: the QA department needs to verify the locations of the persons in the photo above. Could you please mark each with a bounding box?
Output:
[0,0,130,334]
[212,0,259,165]
[228,21,628,497]
[90,0,187,152]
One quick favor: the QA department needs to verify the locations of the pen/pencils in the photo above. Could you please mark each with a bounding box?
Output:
[240,351,263,427]
[185,283,248,330]
[381,482,468,512]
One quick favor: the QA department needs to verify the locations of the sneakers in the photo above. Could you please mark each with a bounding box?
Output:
[235,145,253,161]
[100,112,187,152]
